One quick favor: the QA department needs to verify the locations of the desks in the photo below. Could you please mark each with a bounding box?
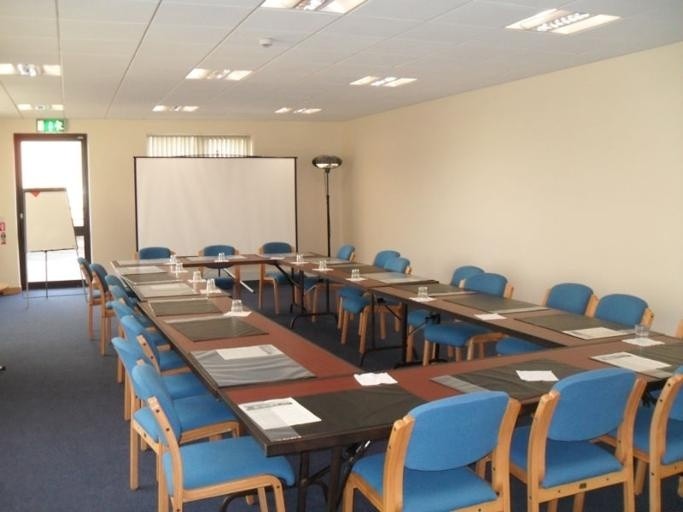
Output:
[112,253,682,512]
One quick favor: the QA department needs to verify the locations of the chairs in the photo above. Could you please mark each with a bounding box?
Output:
[132,359,296,512]
[108,285,171,384]
[342,256,413,352]
[302,245,354,324]
[341,391,512,512]
[197,244,240,304]
[593,365,683,512]
[112,300,175,420]
[119,316,209,450]
[104,274,145,384]
[89,263,138,356]
[422,273,513,368]
[134,247,175,260]
[590,293,655,331]
[78,257,114,343]
[258,241,297,316]
[496,283,598,358]
[338,250,400,329]
[405,264,485,369]
[508,367,647,512]
[111,338,241,489]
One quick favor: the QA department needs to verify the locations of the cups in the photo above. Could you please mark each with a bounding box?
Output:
[176,263,184,271]
[319,260,327,270]
[231,299,243,313]
[193,271,201,281]
[297,254,304,263]
[219,252,226,261]
[634,325,650,340]
[207,279,215,291]
[170,255,177,262]
[351,268,360,279]
[418,287,428,298]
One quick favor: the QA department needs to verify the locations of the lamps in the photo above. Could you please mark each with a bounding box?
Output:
[312,155,343,258]
